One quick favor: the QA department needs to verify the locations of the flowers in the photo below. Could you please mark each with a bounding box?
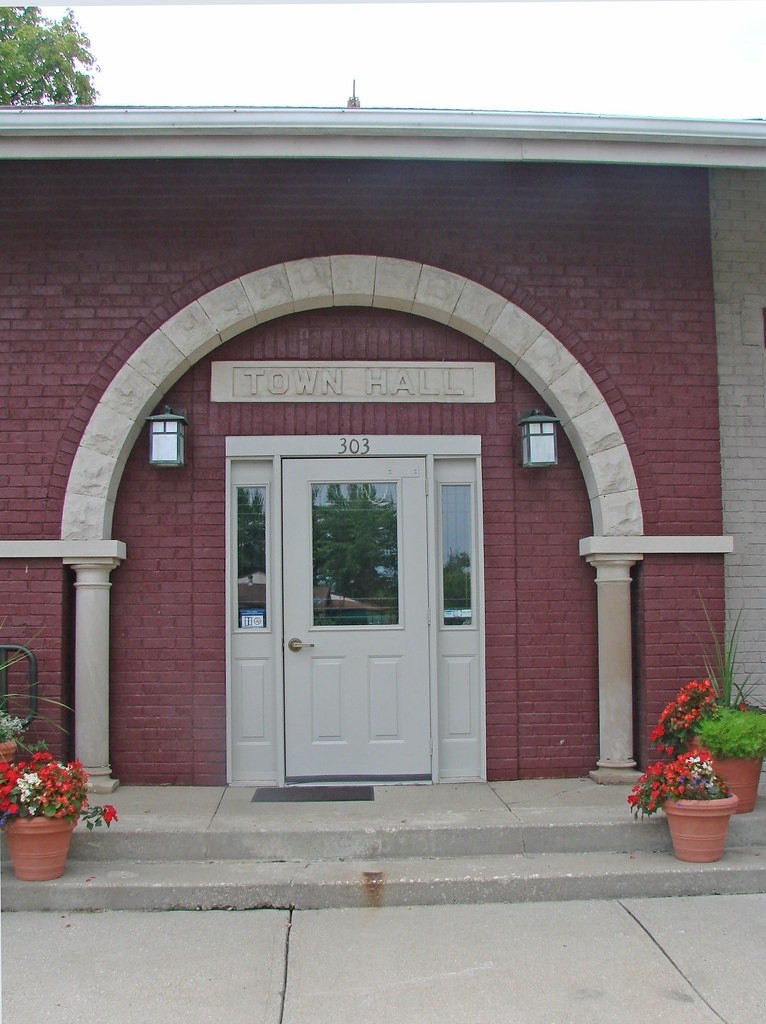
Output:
[626,749,727,820]
[0,750,120,828]
[646,586,766,761]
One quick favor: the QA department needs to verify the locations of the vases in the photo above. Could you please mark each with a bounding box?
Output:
[712,758,764,813]
[3,817,74,881]
[660,791,740,863]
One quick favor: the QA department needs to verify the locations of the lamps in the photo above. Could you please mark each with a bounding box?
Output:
[146,405,189,467]
[515,408,562,469]
